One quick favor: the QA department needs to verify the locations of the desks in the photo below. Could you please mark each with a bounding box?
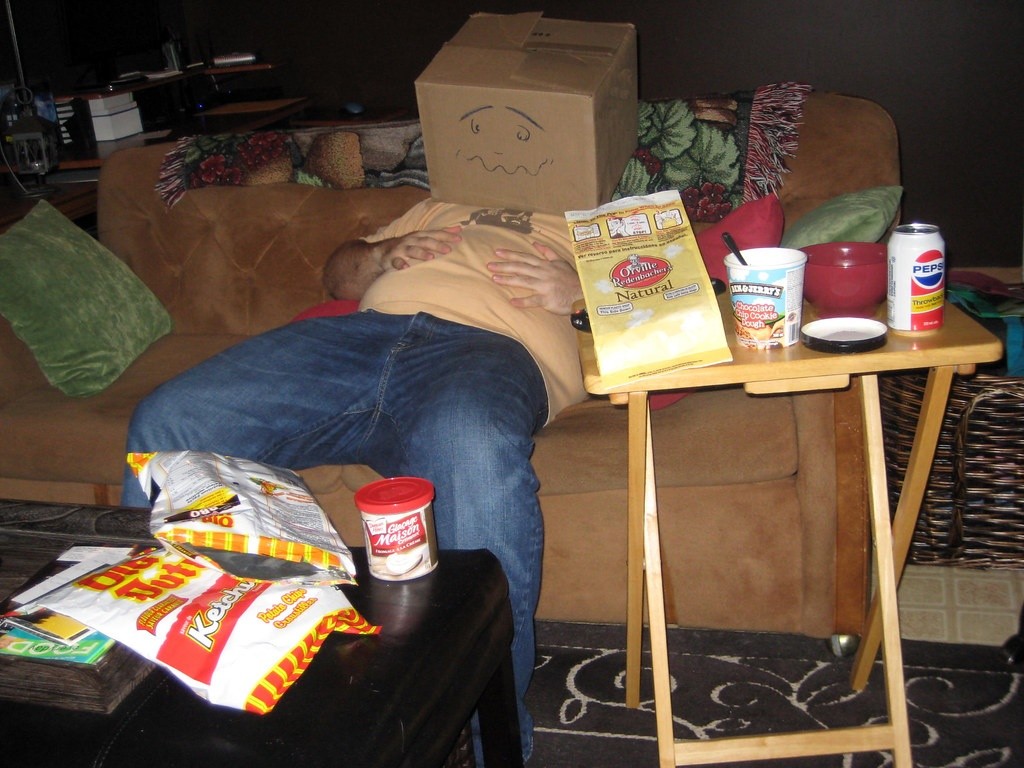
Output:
[0,60,314,235]
[572,285,1003,768]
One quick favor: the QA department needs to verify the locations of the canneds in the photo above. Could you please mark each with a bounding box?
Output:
[887,223,944,336]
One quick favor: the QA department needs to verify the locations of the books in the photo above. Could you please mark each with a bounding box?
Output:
[0,542,191,714]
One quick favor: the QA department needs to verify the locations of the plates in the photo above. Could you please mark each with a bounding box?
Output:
[801,317,888,353]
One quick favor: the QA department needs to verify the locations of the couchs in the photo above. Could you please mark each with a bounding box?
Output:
[0,92,900,640]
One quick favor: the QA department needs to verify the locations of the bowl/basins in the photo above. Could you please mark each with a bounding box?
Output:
[797,242,889,319]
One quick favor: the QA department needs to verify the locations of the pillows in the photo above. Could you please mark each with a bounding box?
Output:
[649,194,785,411]
[0,199,175,398]
[780,184,904,251]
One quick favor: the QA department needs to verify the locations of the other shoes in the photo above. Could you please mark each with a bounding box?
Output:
[521,732,534,764]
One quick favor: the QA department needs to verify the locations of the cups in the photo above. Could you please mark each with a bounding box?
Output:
[724,247,808,350]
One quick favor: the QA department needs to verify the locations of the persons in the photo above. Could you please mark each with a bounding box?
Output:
[120,200,584,768]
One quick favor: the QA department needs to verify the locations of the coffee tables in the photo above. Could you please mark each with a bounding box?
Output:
[0,544,525,768]
